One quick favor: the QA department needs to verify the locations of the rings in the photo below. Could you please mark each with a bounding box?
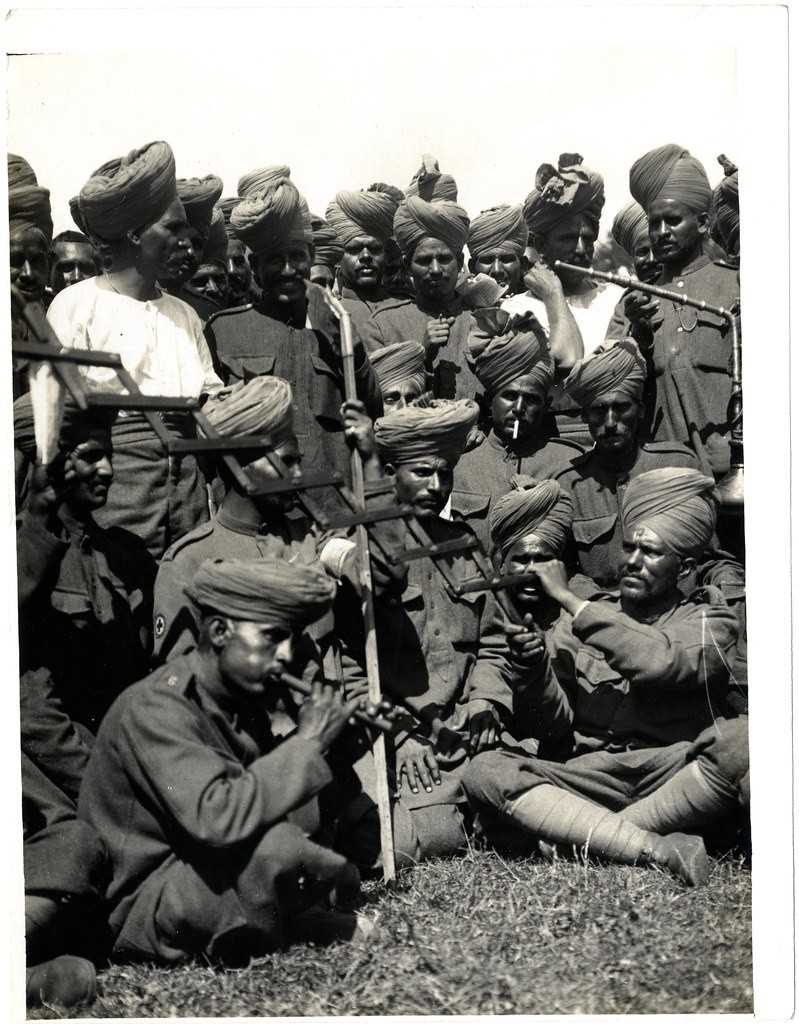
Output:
[351,426,355,436]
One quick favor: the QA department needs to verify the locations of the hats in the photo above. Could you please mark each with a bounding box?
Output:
[7,144,739,298]
[14,383,123,456]
[621,467,722,556]
[373,397,479,464]
[367,339,427,396]
[565,335,647,407]
[490,470,572,555]
[462,310,555,392]
[197,375,294,460]
[182,557,336,629]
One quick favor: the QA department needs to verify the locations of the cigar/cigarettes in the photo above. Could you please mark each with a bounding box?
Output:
[440,314,442,324]
[513,420,519,440]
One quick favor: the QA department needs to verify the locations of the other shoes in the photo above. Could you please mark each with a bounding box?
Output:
[475,824,548,860]
[26,954,97,1008]
[334,863,362,902]
[303,908,357,942]
[641,832,709,887]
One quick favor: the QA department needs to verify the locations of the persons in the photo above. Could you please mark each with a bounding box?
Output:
[460,465,750,883]
[7,135,750,1021]
[79,558,407,963]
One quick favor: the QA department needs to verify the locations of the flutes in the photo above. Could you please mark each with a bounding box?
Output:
[276,671,394,731]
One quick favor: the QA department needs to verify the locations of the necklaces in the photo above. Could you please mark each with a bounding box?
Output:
[105,272,158,301]
